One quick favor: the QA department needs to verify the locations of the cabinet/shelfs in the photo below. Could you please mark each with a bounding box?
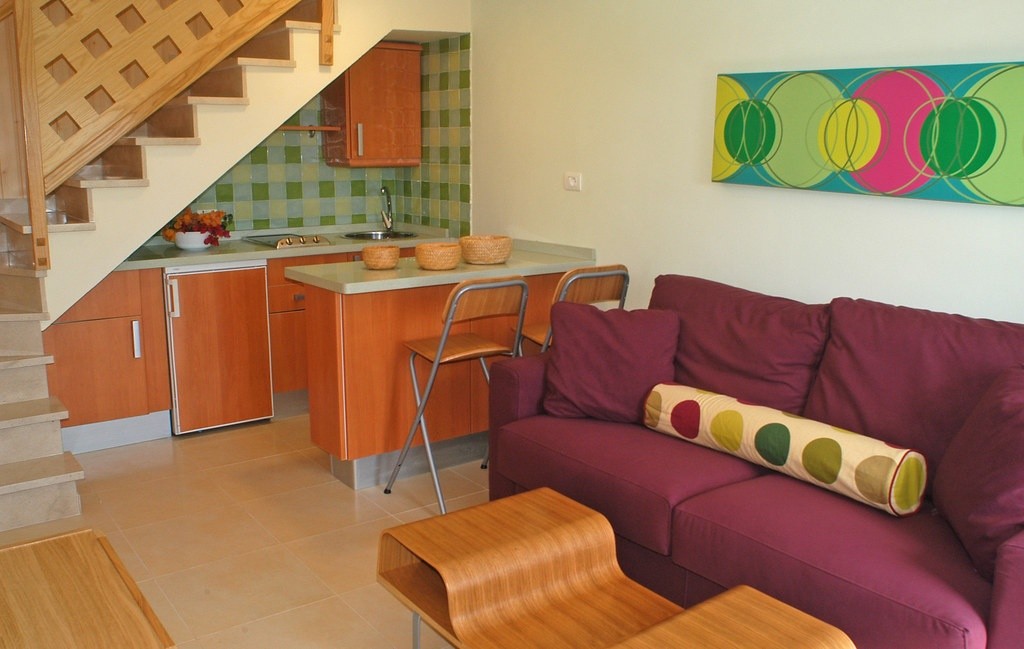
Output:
[40,270,150,430]
[323,42,424,168]
[264,247,416,395]
[162,266,276,435]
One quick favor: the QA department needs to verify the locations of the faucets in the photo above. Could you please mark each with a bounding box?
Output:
[379,186,393,230]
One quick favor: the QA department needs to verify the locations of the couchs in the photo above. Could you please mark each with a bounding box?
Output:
[490,351,1024,648]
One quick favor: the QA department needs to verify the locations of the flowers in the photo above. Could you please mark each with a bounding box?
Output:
[161,206,234,247]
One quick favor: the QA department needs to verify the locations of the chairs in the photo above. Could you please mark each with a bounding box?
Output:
[512,264,631,357]
[381,275,530,517]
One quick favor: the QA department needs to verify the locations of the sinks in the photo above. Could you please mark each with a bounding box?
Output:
[341,230,418,241]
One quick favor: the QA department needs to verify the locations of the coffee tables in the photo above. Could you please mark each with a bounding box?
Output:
[375,486,857,649]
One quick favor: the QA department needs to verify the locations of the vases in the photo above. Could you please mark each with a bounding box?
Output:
[174,231,213,251]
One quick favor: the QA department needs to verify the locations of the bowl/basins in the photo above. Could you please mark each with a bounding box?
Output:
[175,232,211,249]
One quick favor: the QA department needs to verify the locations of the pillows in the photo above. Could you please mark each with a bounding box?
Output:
[540,300,682,423]
[802,298,1023,498]
[926,359,1024,581]
[643,382,925,517]
[647,273,832,414]
